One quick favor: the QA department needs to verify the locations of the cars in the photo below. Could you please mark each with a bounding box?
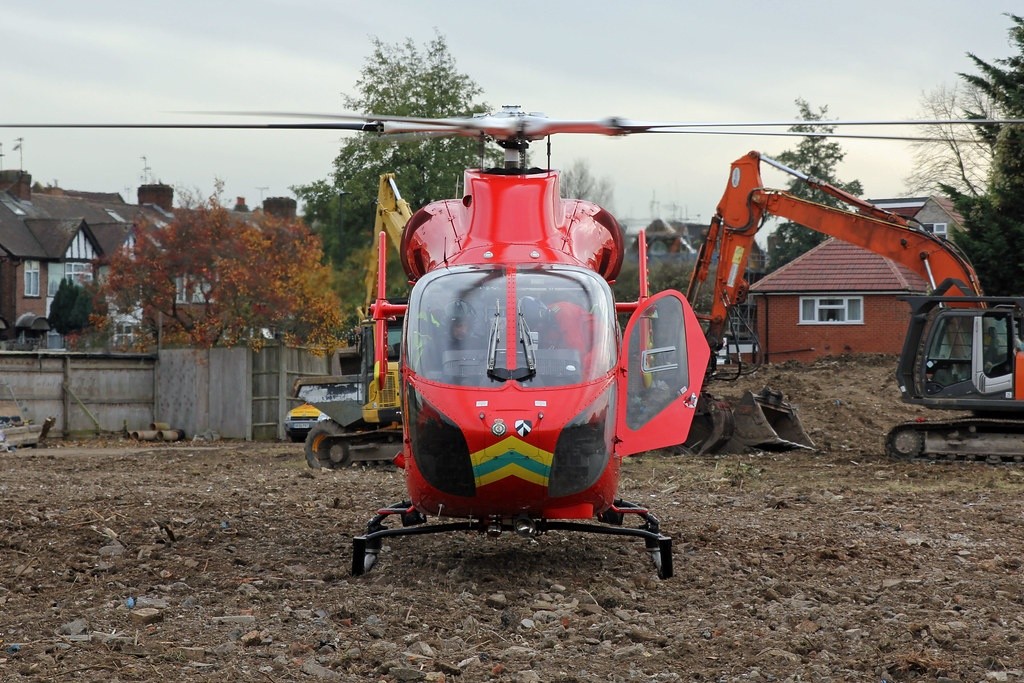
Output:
[283,401,331,443]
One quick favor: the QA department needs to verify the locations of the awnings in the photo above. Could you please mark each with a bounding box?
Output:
[17,315,51,331]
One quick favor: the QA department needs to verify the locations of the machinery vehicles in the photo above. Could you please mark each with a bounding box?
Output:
[643,150,1023,463]
[291,173,414,469]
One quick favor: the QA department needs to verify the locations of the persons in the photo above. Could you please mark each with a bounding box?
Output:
[518,296,612,388]
[418,298,487,385]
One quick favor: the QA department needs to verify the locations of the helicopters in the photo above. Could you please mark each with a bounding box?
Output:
[1,104,1024,579]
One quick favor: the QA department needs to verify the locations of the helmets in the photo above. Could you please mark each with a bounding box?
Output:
[444,298,478,340]
[518,296,549,332]
